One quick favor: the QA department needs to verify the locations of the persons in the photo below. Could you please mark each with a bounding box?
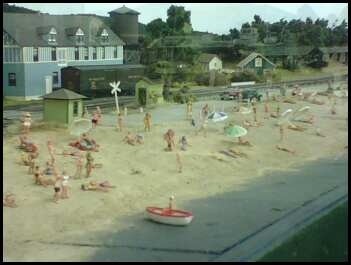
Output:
[4,79,346,205]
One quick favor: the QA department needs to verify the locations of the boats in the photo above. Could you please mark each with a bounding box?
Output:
[145,197,194,226]
